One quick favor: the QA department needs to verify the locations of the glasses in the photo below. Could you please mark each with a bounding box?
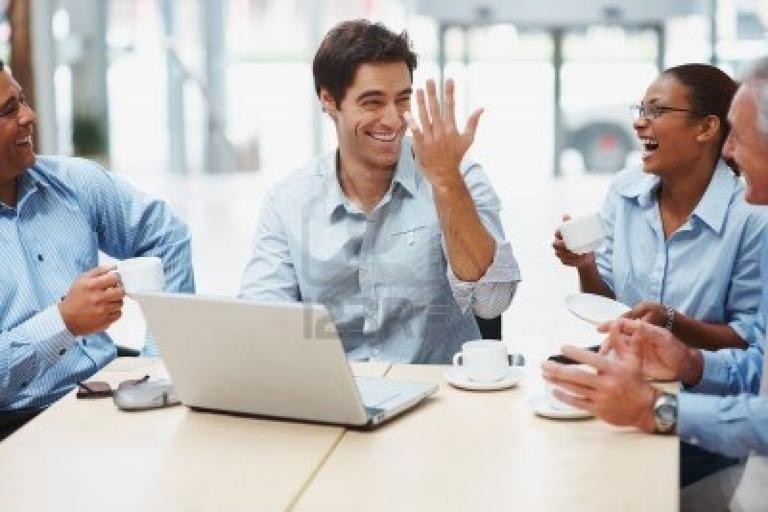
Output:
[75,372,150,397]
[627,104,690,122]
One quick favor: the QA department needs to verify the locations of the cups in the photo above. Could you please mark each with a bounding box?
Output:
[545,362,597,402]
[114,255,166,297]
[560,213,603,255]
[453,338,508,382]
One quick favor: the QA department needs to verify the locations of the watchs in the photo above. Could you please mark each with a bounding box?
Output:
[653,392,677,432]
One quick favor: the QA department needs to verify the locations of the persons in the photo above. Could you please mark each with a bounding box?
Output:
[553,64,767,349]
[540,56,768,512]
[1,60,195,441]
[235,18,521,364]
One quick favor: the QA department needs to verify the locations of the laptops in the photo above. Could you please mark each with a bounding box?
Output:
[135,291,438,432]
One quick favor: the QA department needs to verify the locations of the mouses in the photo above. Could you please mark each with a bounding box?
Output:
[113,380,179,412]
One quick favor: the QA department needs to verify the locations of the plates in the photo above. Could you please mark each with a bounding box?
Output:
[567,293,639,326]
[536,404,595,421]
[448,377,516,391]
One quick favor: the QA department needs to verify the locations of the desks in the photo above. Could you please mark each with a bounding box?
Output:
[0,356,392,508]
[291,364,680,512]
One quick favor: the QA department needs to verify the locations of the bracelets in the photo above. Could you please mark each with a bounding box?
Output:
[666,307,675,330]
